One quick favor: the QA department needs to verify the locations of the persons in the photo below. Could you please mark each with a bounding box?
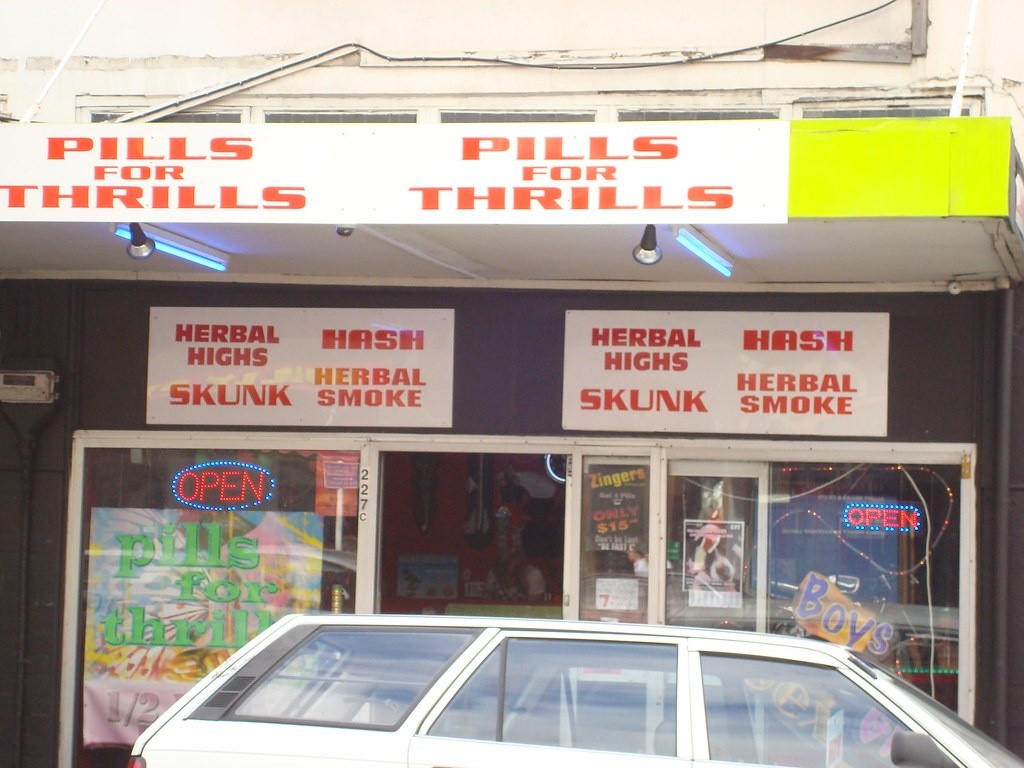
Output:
[626,549,649,578]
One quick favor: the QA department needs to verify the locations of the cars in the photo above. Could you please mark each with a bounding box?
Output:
[128,609,1024,768]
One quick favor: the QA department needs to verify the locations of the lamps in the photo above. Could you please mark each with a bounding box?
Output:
[126,222,155,260]
[632,224,663,265]
[671,224,736,276]
[108,222,231,272]
